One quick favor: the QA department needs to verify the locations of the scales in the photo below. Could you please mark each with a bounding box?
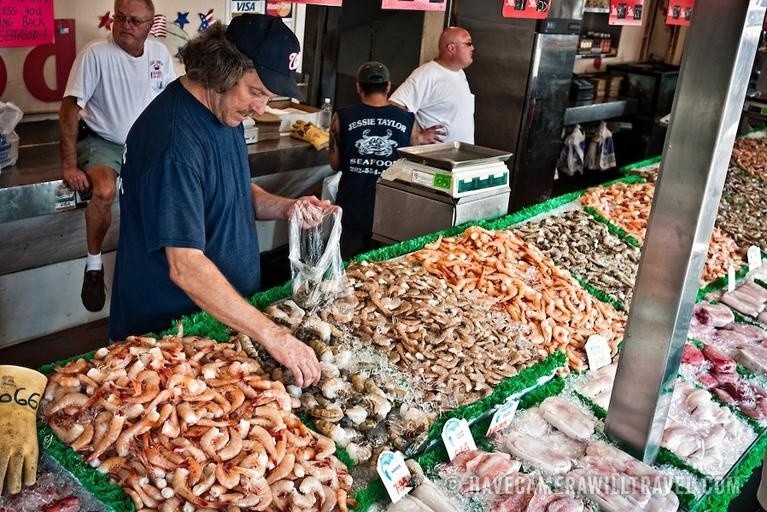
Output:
[379,140,515,198]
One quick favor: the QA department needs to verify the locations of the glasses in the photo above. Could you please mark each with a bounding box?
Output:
[113,13,152,28]
[454,41,471,46]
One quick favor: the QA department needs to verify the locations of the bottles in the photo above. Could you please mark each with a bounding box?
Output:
[317,97,334,132]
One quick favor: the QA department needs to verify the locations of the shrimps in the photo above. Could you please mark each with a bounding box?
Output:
[37,181,655,512]
[697,136,767,287]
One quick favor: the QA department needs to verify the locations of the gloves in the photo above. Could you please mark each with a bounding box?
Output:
[0,364,48,496]
[290,120,331,150]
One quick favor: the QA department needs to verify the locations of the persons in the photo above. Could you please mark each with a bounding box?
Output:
[384,25,477,151]
[56,1,179,314]
[103,9,344,391]
[327,61,416,262]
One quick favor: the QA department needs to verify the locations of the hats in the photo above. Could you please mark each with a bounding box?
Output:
[226,12,306,103]
[357,62,391,83]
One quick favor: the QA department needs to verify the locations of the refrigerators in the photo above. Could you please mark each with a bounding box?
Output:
[450,0,587,213]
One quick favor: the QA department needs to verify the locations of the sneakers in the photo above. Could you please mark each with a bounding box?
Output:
[81,263,105,312]
